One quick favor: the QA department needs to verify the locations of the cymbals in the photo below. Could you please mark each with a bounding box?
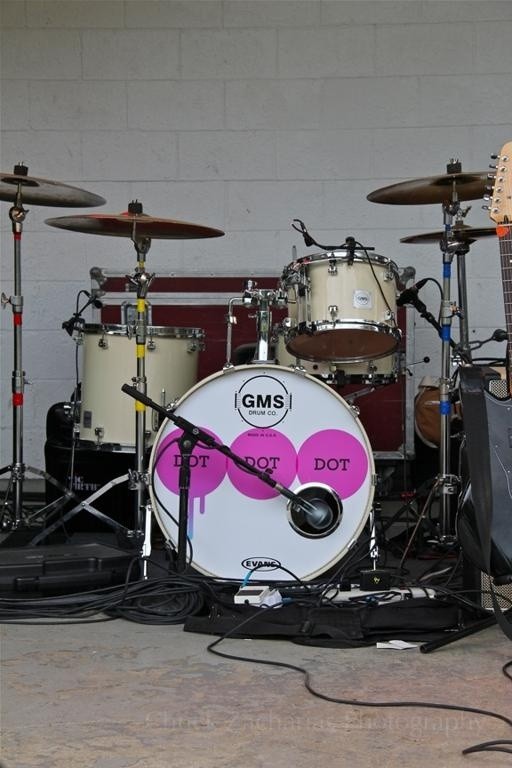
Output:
[45,213,224,238]
[0,173,107,206]
[367,172,496,205]
[400,227,496,242]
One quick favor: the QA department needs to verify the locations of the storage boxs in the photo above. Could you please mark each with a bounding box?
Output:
[84,263,421,464]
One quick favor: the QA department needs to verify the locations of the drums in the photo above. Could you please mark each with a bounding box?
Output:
[281,252,400,363]
[274,325,399,385]
[78,324,205,454]
[147,364,376,583]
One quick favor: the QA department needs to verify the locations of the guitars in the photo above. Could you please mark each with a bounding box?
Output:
[455,141,512,587]
